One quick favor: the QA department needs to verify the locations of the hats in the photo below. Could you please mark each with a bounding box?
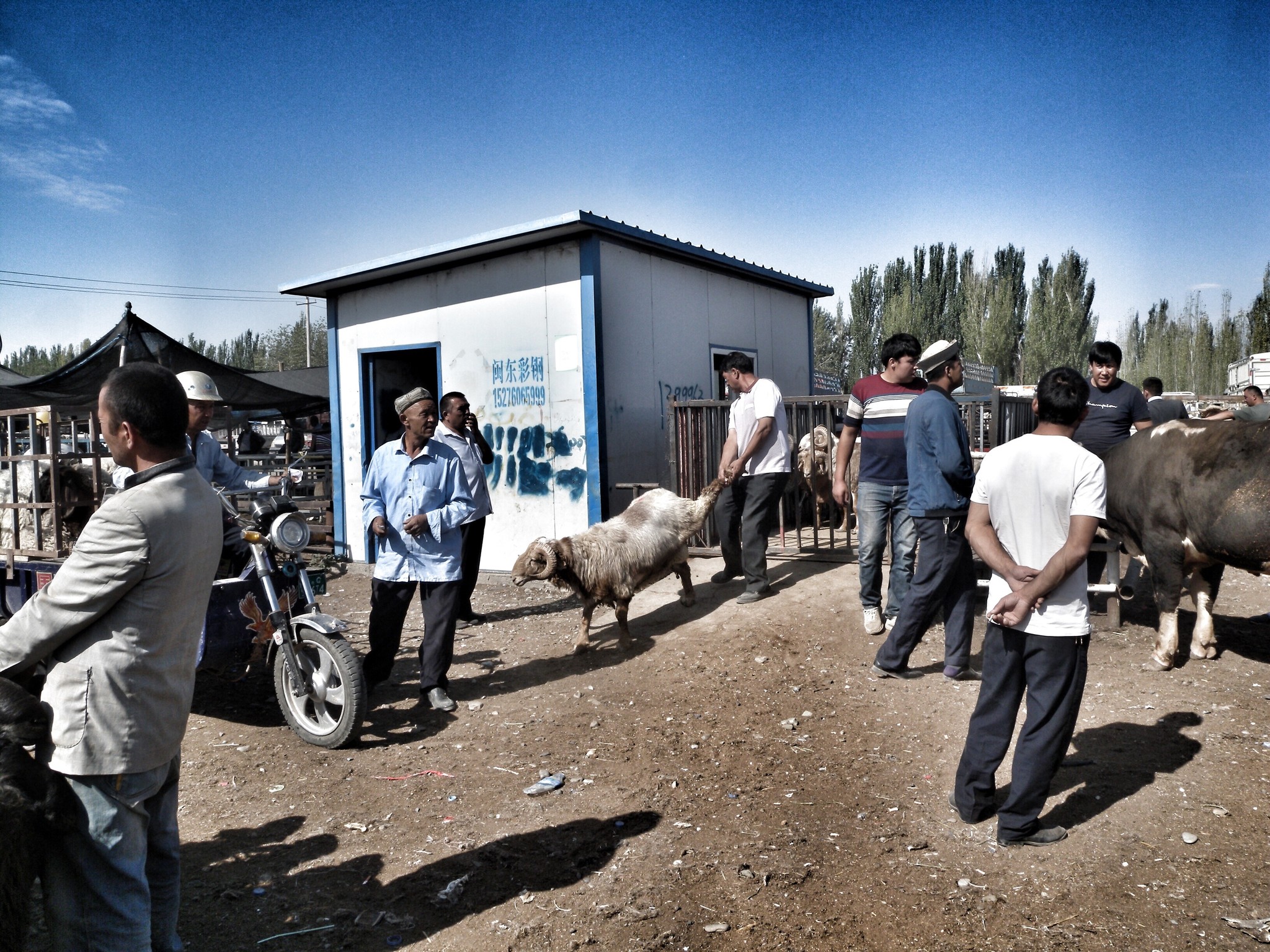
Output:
[914,338,959,374]
[393,387,434,417]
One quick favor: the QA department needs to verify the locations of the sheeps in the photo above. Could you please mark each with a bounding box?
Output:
[0,441,119,556]
[511,470,733,656]
[785,423,859,534]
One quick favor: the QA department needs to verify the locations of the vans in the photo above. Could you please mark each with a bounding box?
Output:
[269,428,308,455]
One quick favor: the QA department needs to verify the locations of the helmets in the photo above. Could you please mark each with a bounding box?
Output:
[176,371,224,401]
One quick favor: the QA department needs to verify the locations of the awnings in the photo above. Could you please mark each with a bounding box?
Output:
[0,301,330,412]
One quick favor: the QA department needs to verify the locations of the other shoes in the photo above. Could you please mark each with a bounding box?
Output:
[459,612,487,621]
[454,617,468,629]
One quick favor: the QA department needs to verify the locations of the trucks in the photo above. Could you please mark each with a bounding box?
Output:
[1160,391,1199,419]
[1222,352,1270,411]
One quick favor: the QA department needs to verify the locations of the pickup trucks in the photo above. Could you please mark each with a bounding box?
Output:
[219,434,239,456]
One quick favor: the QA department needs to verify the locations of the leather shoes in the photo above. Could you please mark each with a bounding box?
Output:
[711,570,744,584]
[736,586,772,604]
[996,822,1067,847]
[421,684,456,712]
[871,658,925,679]
[948,788,978,824]
[942,666,981,681]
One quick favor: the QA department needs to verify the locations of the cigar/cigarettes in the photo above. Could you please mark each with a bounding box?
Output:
[476,415,484,419]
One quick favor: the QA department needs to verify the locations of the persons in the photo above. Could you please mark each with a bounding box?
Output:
[360,387,474,710]
[832,333,927,634]
[713,351,792,604]
[112,371,298,493]
[1143,377,1189,425]
[871,340,983,680]
[0,361,223,952]
[237,422,266,466]
[1191,386,1270,422]
[430,392,495,630]
[279,412,332,480]
[1072,341,1154,616]
[954,365,1107,848]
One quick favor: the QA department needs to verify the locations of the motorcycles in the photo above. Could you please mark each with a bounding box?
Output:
[0,428,369,750]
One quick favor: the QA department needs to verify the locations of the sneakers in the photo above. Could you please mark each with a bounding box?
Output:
[861,605,897,636]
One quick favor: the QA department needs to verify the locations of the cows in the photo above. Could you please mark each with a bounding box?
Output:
[1102,418,1270,672]
